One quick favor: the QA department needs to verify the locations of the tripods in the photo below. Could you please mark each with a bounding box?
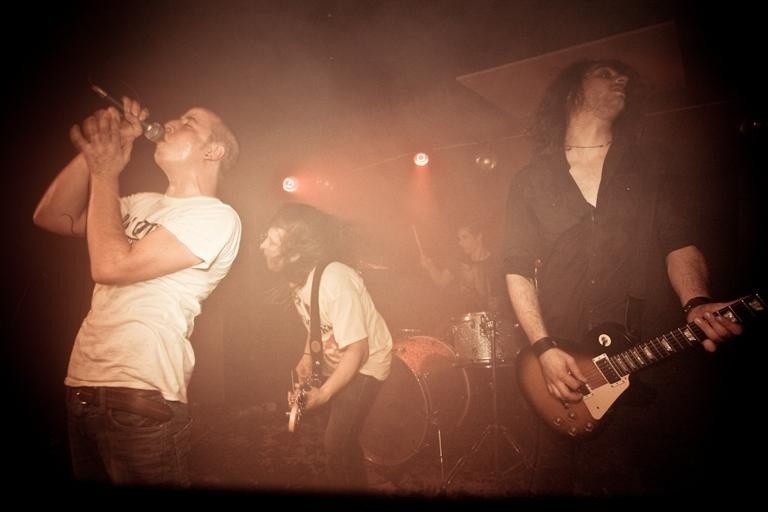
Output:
[431,306,549,496]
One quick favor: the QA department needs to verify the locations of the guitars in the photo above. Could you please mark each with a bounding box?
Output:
[287,357,325,434]
[514,291,761,440]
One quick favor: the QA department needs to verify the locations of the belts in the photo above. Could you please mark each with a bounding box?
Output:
[66,383,174,424]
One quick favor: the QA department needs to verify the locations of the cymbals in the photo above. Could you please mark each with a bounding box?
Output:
[360,261,389,270]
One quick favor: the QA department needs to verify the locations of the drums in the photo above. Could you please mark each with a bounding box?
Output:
[450,312,519,369]
[358,335,471,467]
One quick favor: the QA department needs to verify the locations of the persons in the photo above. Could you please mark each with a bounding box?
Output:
[416,212,506,315]
[31,94,243,490]
[502,60,750,499]
[257,200,398,493]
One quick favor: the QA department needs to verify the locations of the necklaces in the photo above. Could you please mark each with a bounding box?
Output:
[564,141,613,151]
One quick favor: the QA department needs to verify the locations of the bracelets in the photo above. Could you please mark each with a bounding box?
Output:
[530,335,559,357]
[680,294,717,313]
[303,352,312,355]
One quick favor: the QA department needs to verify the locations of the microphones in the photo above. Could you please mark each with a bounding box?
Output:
[90,83,165,143]
